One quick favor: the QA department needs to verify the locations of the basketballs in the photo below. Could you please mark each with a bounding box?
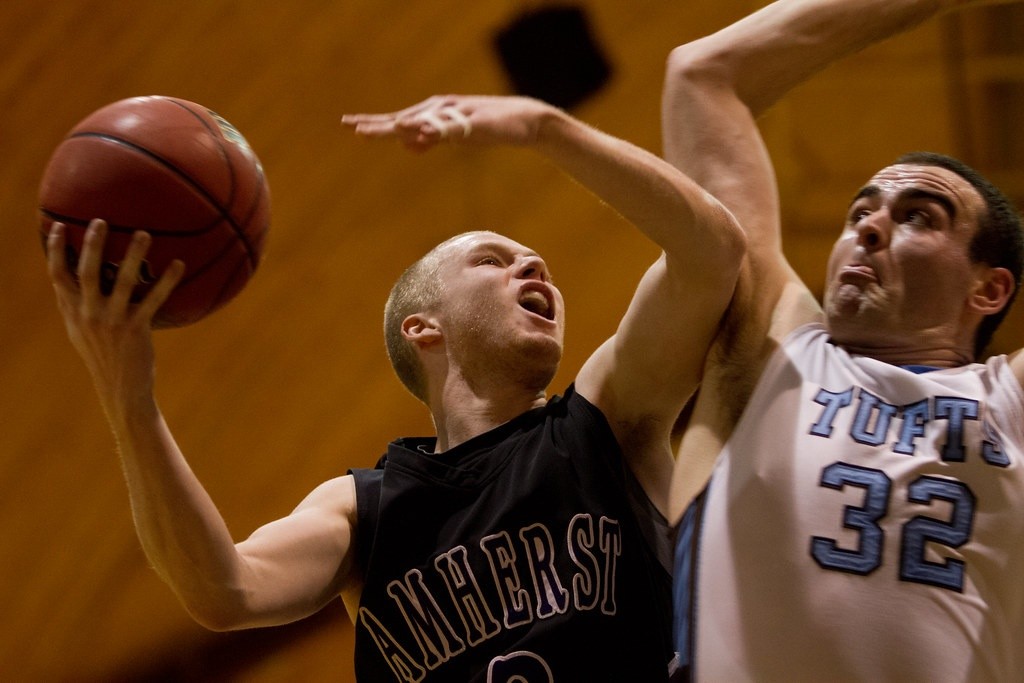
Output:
[34,94,274,332]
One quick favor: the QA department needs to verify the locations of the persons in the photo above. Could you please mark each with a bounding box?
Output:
[47,95,749,683]
[660,0,1024,683]
